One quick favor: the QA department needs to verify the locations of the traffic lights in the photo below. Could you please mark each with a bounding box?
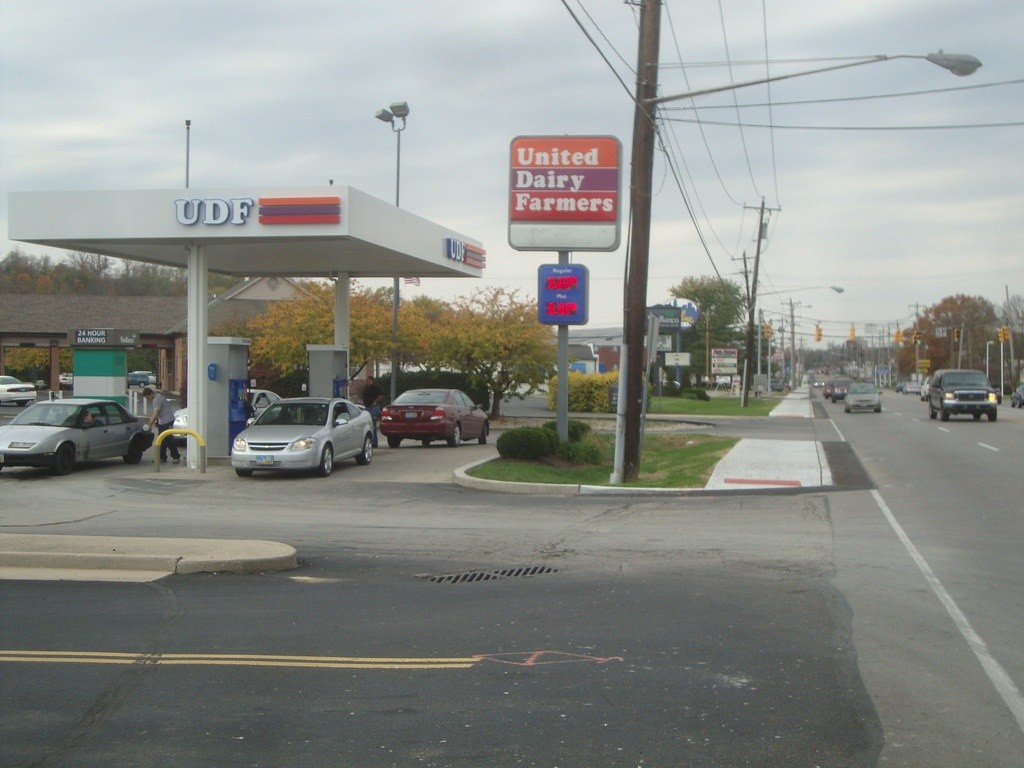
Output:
[815,327,823,341]
[954,328,961,342]
[763,324,773,340]
[997,328,1004,342]
[913,331,922,345]
[1003,327,1010,341]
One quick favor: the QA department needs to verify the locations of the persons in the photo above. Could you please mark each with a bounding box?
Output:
[142,387,181,463]
[364,376,382,416]
[83,412,103,425]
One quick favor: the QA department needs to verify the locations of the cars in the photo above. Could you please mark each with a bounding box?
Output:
[231,397,374,480]
[0,399,155,477]
[56,373,73,389]
[1010,384,1024,408]
[379,388,492,447]
[920,376,932,402]
[811,374,855,403]
[173,388,284,445]
[845,382,883,414]
[903,382,923,394]
[896,379,906,392]
[0,375,38,405]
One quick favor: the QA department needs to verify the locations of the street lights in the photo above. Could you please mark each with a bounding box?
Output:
[373,99,410,410]
[985,341,995,381]
[740,286,845,413]
[613,50,984,498]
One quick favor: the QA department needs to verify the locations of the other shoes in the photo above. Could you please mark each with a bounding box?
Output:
[151,457,166,464]
[372,444,378,448]
[172,458,180,463]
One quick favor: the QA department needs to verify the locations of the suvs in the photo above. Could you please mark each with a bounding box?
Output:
[926,367,1000,423]
[126,373,150,389]
